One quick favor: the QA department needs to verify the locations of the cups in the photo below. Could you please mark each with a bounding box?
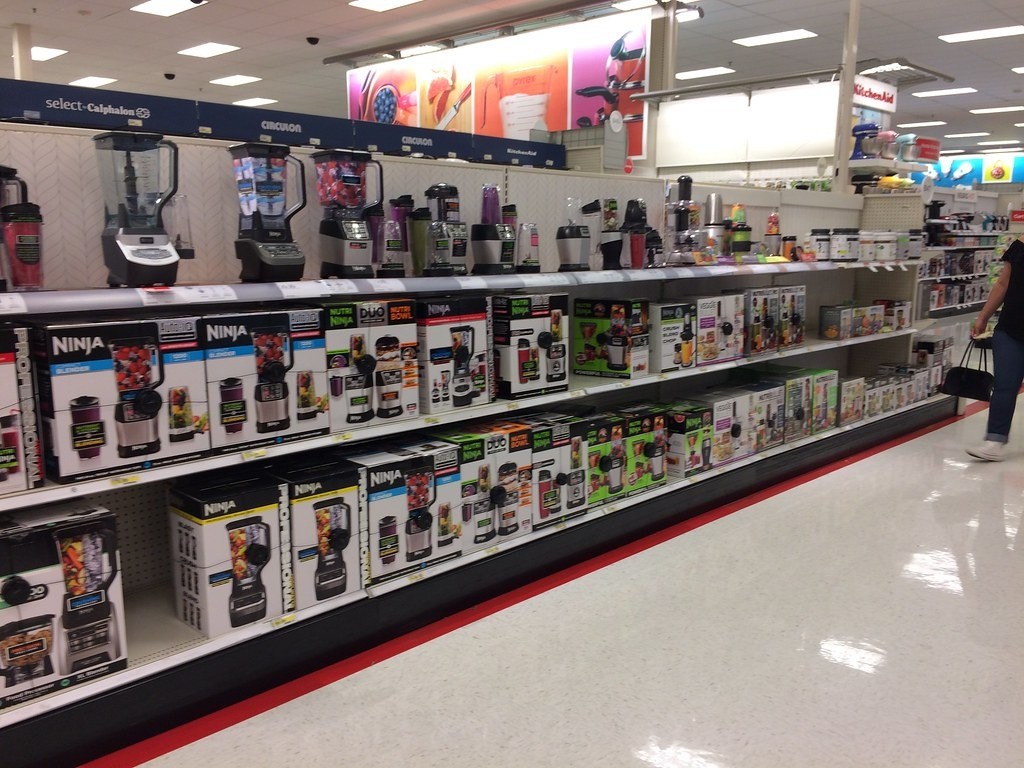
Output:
[372,85,417,126]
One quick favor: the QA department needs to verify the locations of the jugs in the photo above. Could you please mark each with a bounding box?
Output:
[480,65,554,141]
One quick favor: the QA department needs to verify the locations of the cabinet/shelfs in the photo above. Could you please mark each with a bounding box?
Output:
[0,124,1024,768]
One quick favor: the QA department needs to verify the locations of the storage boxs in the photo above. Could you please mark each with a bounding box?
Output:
[0,286,942,710]
[919,237,997,310]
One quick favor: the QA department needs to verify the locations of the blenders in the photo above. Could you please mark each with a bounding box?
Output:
[0,312,942,680]
[0,123,954,293]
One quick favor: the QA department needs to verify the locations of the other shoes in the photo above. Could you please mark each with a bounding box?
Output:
[965,441,1007,462]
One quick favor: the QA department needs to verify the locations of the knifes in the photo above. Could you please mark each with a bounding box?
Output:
[434,83,471,130]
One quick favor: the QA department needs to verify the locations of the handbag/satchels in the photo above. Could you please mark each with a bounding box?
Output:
[938,339,994,402]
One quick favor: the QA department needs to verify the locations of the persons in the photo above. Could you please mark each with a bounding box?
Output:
[964,235,1024,462]
